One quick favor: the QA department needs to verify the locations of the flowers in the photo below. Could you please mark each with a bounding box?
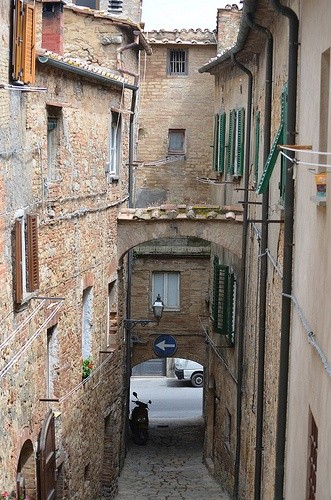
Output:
[82,355,94,379]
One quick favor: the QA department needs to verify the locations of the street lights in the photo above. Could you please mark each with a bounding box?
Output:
[123,294,164,454]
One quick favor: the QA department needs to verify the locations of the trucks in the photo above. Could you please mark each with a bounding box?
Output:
[173,357,204,387]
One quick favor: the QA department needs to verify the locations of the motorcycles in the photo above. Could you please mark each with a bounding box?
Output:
[130,391,152,446]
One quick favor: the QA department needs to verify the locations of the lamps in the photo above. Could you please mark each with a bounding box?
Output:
[153,293,165,323]
